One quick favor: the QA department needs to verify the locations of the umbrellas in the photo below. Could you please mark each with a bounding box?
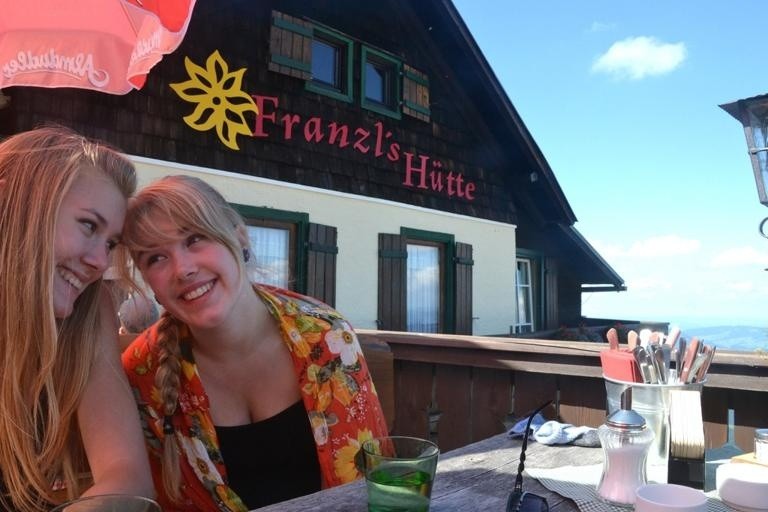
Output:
[0,0,195,94]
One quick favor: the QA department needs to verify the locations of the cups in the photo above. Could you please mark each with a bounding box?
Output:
[636,483,707,511]
[48,493,161,511]
[359,434,441,511]
[715,462,768,512]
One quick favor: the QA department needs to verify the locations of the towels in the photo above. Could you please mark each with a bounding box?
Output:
[508,413,607,448]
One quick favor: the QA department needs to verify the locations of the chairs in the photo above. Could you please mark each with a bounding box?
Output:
[355,334,394,434]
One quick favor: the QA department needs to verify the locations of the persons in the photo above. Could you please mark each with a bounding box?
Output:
[0,125,156,512]
[118,176,397,512]
[119,295,159,334]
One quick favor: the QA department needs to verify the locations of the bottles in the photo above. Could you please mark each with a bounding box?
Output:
[753,428,768,461]
[594,387,654,508]
